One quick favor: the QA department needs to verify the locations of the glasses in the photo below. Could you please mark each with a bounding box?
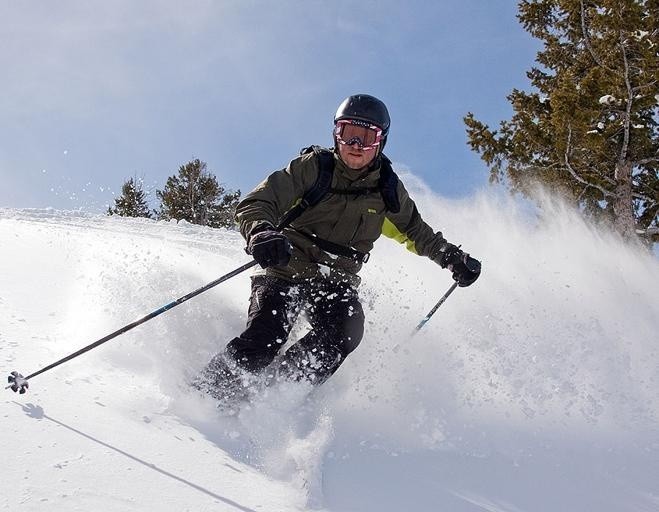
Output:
[333,121,382,152]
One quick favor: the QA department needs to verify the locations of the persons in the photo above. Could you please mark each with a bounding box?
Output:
[206,93,484,410]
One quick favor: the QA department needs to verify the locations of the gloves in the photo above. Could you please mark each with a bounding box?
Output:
[248,219,294,270]
[438,243,483,288]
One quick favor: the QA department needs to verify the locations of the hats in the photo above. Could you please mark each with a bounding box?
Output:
[333,92,391,137]
